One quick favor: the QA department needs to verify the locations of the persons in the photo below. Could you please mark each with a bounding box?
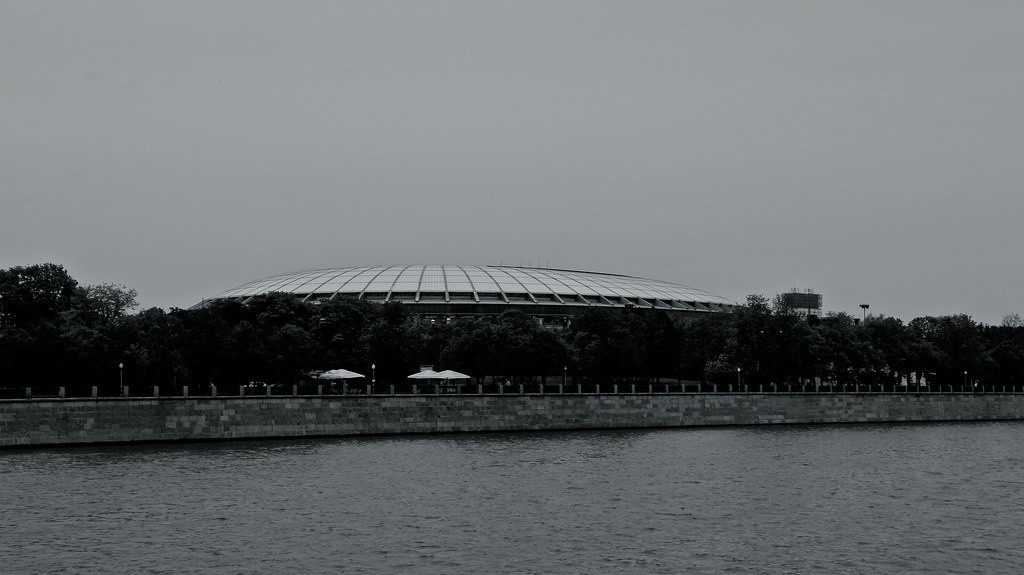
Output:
[503,378,513,389]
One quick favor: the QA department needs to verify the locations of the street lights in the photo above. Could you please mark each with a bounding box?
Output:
[565,366,569,393]
[371,364,376,393]
[737,367,741,391]
[964,371,968,391]
[119,362,123,396]
